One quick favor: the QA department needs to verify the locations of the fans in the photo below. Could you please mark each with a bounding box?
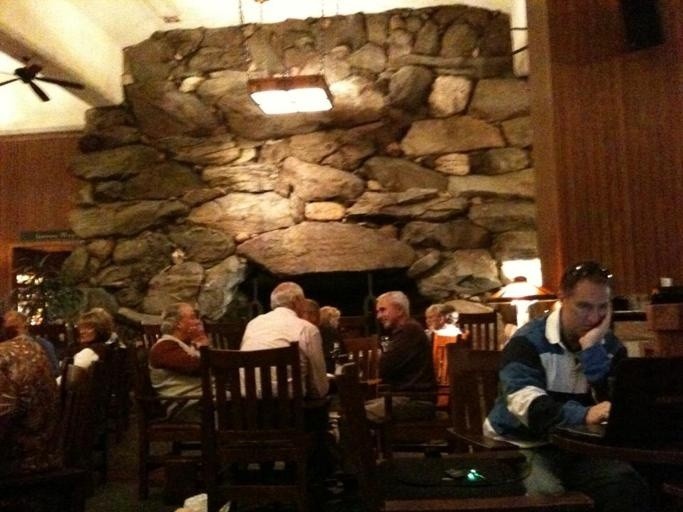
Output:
[0,56,85,102]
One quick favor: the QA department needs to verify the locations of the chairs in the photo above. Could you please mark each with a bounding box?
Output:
[331,362,527,509]
[332,312,514,454]
[27,340,126,501]
[121,314,243,496]
[196,339,308,507]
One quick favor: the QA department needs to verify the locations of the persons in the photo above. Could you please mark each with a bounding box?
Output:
[147,301,231,430]
[319,305,348,374]
[0,307,128,486]
[362,290,439,421]
[482,261,628,497]
[424,304,463,343]
[299,298,321,325]
[238,280,337,482]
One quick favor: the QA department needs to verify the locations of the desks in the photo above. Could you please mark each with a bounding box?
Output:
[550,432,683,512]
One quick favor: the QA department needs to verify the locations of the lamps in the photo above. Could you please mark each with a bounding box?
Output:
[239,0,333,115]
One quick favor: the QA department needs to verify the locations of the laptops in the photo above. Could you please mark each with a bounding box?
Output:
[551,357,681,449]
